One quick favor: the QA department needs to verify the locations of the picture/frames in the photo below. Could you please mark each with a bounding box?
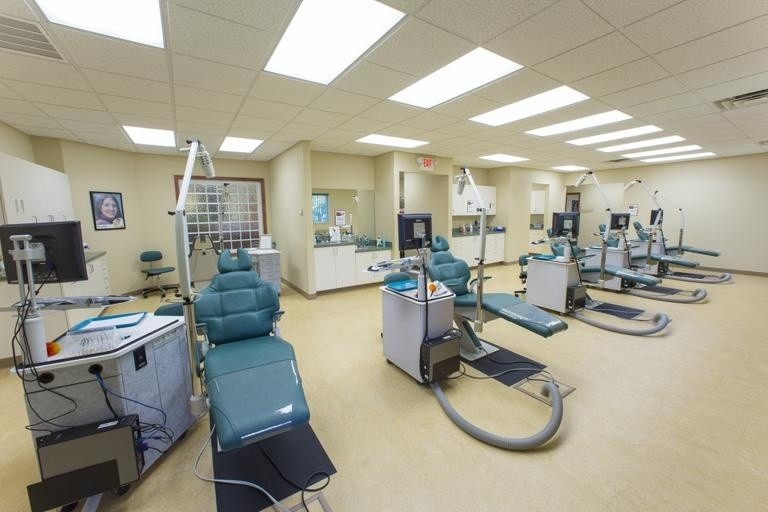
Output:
[90,191,127,231]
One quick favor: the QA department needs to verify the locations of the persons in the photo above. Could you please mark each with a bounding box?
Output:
[95,194,122,225]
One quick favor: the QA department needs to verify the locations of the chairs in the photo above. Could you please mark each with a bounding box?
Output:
[193,247,311,454]
[513,254,531,297]
[551,240,662,292]
[381,272,411,338]
[599,223,700,268]
[633,220,721,258]
[154,302,212,364]
[428,235,569,338]
[139,250,182,300]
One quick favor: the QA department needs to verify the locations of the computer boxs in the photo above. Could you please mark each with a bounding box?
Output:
[566,284,586,311]
[621,268,637,288]
[36,413,145,488]
[421,332,460,383]
[658,260,669,273]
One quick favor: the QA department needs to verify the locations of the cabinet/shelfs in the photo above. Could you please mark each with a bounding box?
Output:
[0,257,111,366]
[452,184,497,216]
[355,250,391,286]
[0,151,76,224]
[315,242,358,292]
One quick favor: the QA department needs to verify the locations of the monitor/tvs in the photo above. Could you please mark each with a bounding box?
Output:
[259,234,272,249]
[611,213,630,230]
[0,221,88,285]
[650,210,663,225]
[397,213,432,250]
[552,212,580,237]
